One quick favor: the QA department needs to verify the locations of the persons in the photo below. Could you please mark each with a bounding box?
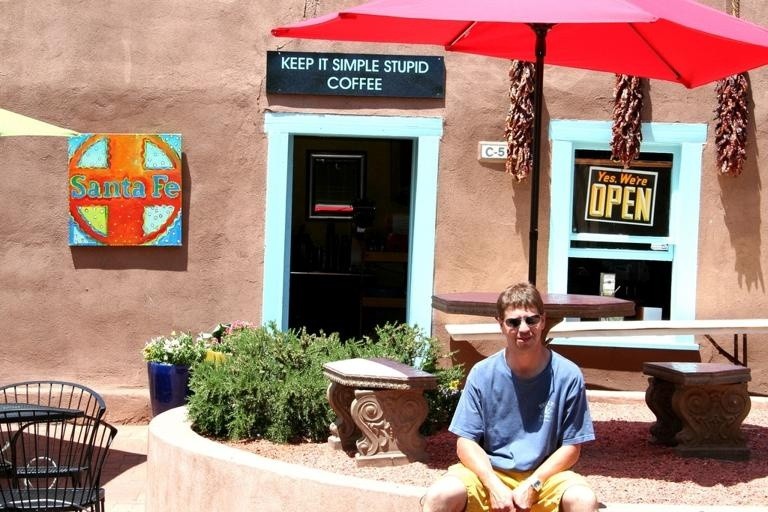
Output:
[417,281,609,511]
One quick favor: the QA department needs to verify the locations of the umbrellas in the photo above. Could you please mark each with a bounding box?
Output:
[268,2,768,294]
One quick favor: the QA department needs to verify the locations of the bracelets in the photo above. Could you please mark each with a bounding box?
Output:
[525,477,544,493]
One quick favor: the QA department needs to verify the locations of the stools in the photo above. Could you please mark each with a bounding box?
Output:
[641,360,755,464]
[321,354,443,470]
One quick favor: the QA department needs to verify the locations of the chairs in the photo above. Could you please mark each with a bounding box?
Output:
[0,379,118,511]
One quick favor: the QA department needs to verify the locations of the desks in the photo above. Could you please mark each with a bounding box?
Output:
[432,291,635,346]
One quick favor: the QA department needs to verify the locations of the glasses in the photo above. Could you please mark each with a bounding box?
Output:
[504,315,542,327]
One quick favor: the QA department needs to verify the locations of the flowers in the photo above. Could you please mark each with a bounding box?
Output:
[141,329,203,366]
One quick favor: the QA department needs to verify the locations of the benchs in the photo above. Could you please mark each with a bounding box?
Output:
[445,318,768,341]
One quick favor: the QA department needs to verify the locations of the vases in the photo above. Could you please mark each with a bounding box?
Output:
[146,360,193,417]
